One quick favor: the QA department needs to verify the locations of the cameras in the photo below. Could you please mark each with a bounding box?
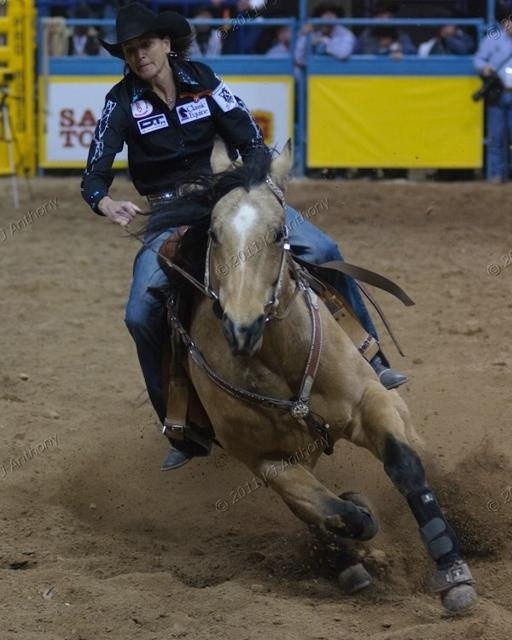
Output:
[472,71,504,102]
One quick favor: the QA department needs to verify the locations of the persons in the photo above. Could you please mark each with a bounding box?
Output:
[78,1,411,473]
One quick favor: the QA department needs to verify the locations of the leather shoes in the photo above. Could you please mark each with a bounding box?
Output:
[369,353,410,392]
[159,442,193,472]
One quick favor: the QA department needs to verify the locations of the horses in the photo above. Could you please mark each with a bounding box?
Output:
[119,135,482,614]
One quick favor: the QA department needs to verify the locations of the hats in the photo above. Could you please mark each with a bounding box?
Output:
[97,2,193,60]
